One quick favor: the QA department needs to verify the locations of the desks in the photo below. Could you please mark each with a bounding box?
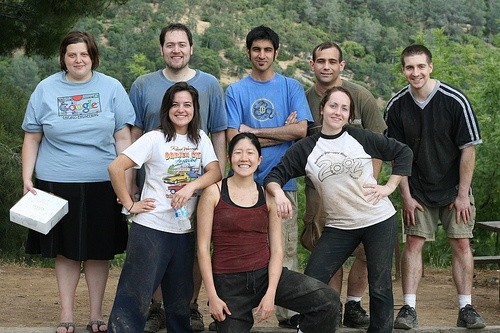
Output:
[476,220,500,237]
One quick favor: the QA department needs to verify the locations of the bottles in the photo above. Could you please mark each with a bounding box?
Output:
[174,204,191,231]
[121,193,140,215]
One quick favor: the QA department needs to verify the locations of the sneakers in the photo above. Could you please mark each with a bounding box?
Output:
[394,305,417,330]
[279,314,301,329]
[188,303,204,332]
[342,300,370,328]
[144,303,166,333]
[458,304,486,329]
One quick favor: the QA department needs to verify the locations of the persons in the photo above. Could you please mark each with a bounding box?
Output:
[223,24,315,328]
[127,24,228,333]
[21,31,136,333]
[301,42,388,328]
[384,44,486,329]
[197,132,341,333]
[263,86,413,333]
[108,81,220,333]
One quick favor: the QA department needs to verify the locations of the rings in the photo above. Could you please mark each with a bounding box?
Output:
[284,121,288,124]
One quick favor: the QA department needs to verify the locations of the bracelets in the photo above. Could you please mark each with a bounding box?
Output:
[128,201,134,213]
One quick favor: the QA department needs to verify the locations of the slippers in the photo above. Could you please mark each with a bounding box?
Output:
[56,322,75,333]
[86,320,107,333]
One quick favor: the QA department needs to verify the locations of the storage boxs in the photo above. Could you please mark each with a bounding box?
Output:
[9,187,69,235]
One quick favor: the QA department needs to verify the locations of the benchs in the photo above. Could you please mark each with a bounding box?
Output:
[473,255,500,261]
